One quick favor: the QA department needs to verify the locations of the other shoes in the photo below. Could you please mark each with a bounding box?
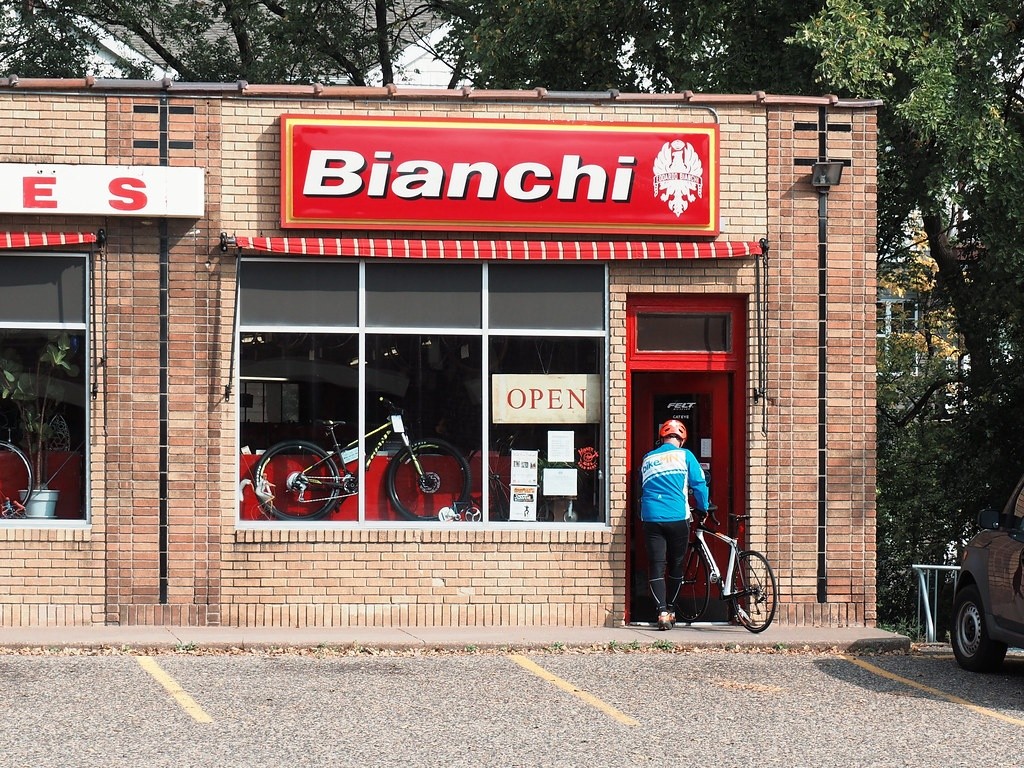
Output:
[658,615,672,630]
[670,612,677,624]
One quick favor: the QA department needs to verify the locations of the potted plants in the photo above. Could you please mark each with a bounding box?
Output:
[0,330,80,519]
[538,448,589,522]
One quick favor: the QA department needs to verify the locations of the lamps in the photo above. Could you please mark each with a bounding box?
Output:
[811,160,844,194]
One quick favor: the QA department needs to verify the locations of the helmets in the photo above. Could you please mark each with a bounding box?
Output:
[659,420,686,439]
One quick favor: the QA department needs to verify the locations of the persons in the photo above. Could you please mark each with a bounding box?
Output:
[639,418,707,635]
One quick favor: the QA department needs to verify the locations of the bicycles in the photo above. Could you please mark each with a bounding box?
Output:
[0,440,34,518]
[438,496,482,522]
[254,396,471,520]
[675,498,778,633]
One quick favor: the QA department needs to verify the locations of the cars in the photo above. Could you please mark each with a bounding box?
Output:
[950,473,1024,669]
